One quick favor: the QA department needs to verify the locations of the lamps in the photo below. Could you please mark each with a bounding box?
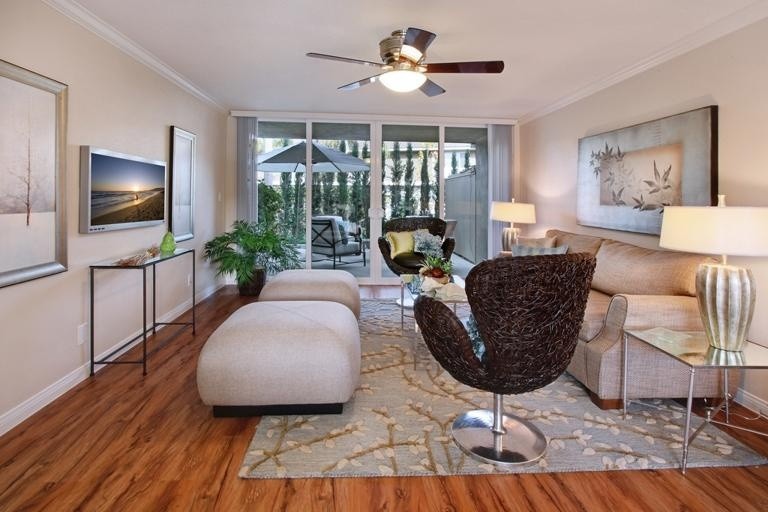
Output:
[489,197,536,251]
[379,71,426,93]
[659,194,768,355]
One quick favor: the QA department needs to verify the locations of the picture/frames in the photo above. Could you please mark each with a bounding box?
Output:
[169,125,195,243]
[0,57,68,295]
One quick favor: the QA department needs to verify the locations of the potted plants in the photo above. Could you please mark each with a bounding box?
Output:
[417,246,454,279]
[205,214,299,297]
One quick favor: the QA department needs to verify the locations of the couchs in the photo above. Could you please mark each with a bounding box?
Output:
[504,230,742,411]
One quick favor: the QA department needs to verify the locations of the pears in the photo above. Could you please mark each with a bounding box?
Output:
[160,231,176,253]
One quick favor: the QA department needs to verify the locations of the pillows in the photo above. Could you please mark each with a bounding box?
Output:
[515,234,559,247]
[466,311,487,361]
[412,229,443,258]
[387,227,430,259]
[509,245,569,256]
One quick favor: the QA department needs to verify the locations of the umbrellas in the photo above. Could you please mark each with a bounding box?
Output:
[255,141,370,173]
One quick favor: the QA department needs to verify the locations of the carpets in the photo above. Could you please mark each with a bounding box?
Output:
[234,300,767,480]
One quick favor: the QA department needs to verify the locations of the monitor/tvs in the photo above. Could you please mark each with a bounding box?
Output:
[81,145,169,233]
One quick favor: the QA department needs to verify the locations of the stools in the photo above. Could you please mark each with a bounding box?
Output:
[257,268,360,320]
[197,299,359,418]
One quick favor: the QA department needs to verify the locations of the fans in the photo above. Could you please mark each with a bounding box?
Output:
[307,25,505,99]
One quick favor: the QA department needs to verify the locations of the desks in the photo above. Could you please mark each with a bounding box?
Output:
[621,323,766,475]
[89,247,195,379]
[394,269,469,372]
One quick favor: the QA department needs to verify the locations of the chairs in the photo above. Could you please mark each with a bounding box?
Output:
[413,252,599,470]
[377,215,454,309]
[311,213,367,270]
